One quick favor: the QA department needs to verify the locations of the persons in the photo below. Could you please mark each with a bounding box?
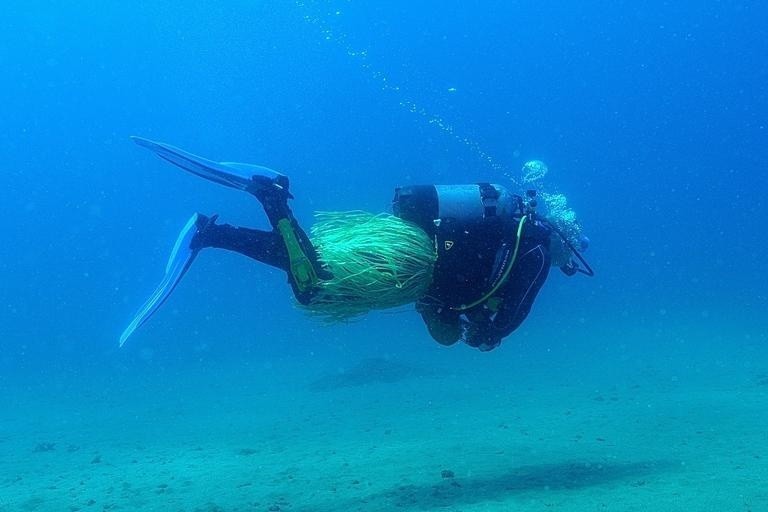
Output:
[119,135,590,354]
